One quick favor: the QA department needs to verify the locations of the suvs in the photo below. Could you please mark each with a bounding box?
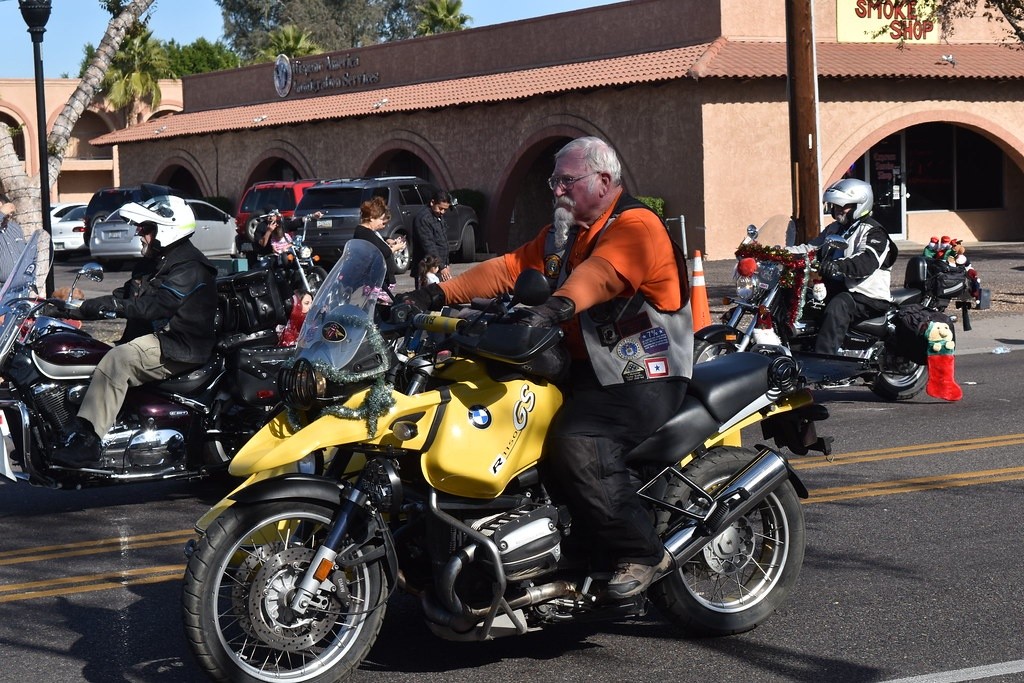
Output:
[235,179,327,247]
[293,177,480,273]
[83,186,202,253]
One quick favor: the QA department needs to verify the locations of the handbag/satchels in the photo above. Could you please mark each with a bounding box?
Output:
[893,304,931,365]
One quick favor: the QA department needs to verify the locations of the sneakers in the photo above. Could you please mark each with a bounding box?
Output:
[608,551,673,599]
[51,429,102,469]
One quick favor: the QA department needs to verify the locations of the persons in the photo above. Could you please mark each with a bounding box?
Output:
[252,209,324,261]
[392,135,693,600]
[40,195,218,471]
[416,255,441,289]
[337,196,407,302]
[278,288,315,346]
[0,194,37,293]
[409,189,452,290]
[270,226,295,254]
[769,178,899,356]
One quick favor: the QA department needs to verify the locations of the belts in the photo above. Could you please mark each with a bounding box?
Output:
[8,286,26,293]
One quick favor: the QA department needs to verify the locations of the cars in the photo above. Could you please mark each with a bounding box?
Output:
[48,203,88,259]
[89,200,237,264]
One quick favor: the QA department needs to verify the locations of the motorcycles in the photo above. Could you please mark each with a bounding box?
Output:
[0,200,355,498]
[694,213,991,402]
[180,238,836,683]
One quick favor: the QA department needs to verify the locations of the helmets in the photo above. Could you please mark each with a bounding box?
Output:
[119,195,196,247]
[823,179,874,220]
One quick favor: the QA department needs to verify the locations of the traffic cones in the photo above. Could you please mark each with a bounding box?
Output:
[689,251,712,330]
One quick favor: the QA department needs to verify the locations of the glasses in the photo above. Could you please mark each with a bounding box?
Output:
[134,225,146,236]
[548,171,599,190]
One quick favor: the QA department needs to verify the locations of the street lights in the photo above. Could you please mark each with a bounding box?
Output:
[18,0,54,301]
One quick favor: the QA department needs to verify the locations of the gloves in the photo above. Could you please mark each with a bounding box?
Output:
[79,296,114,320]
[393,283,445,319]
[499,295,575,328]
[818,260,843,280]
